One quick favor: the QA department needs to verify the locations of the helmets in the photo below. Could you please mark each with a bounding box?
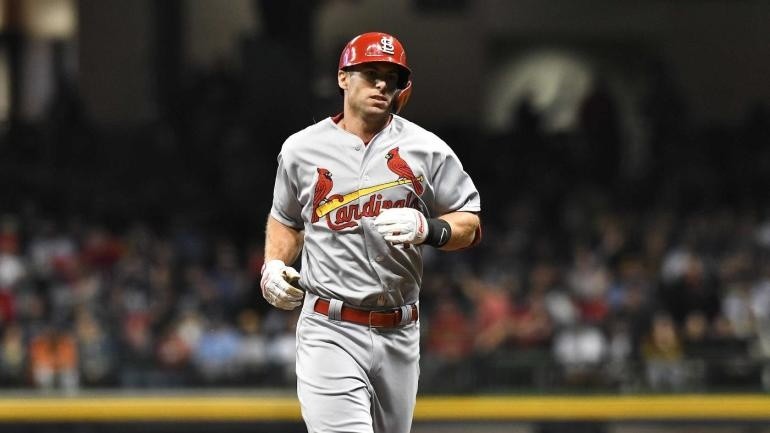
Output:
[338,31,412,75]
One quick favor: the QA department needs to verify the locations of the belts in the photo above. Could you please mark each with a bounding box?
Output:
[314,299,419,326]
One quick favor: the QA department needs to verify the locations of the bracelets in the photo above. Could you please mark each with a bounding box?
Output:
[420,218,450,248]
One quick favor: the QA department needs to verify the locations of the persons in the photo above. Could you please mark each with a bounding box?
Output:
[1,198,770,433]
[261,32,480,433]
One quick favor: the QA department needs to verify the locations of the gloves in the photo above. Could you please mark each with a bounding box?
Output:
[260,260,304,311]
[374,208,428,244]
